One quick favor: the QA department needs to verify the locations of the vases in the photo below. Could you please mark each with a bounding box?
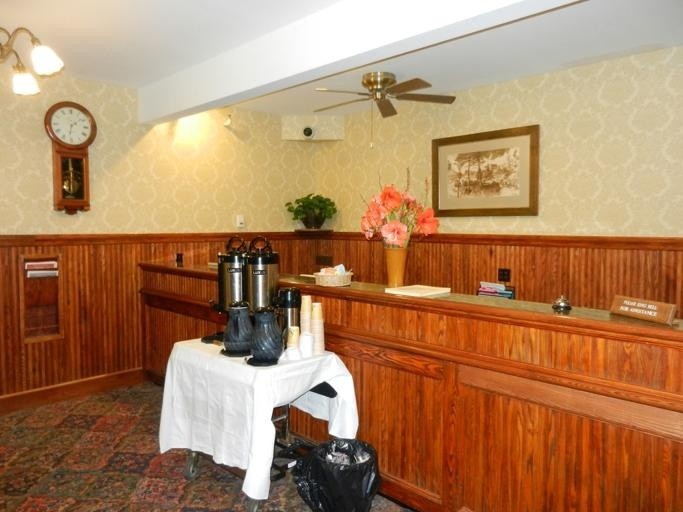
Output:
[386,249,407,288]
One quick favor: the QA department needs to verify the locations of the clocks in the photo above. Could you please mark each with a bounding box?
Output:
[44,100,97,217]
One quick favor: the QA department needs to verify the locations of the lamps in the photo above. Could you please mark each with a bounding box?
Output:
[0,26,65,96]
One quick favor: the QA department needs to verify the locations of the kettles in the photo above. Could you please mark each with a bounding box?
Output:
[221,302,255,357]
[247,308,287,366]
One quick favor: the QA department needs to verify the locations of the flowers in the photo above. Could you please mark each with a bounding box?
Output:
[358,166,441,249]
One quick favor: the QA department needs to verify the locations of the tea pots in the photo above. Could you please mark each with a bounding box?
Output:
[273,287,299,330]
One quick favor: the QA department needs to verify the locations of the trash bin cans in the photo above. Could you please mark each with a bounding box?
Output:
[291,438,380,512]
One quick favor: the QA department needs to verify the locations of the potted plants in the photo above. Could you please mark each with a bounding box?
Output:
[286,193,337,229]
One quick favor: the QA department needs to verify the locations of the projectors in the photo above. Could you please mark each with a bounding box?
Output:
[281,112,346,141]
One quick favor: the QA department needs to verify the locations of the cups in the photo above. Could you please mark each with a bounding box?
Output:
[288,326,299,359]
[300,296,311,332]
[300,334,314,360]
[311,304,324,354]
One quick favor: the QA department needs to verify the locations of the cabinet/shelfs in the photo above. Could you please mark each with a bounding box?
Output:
[136,259,683,512]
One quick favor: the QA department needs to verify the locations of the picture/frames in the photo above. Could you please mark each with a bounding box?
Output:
[431,124,541,216]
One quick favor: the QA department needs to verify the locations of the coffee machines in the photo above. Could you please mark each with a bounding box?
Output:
[201,237,280,350]
